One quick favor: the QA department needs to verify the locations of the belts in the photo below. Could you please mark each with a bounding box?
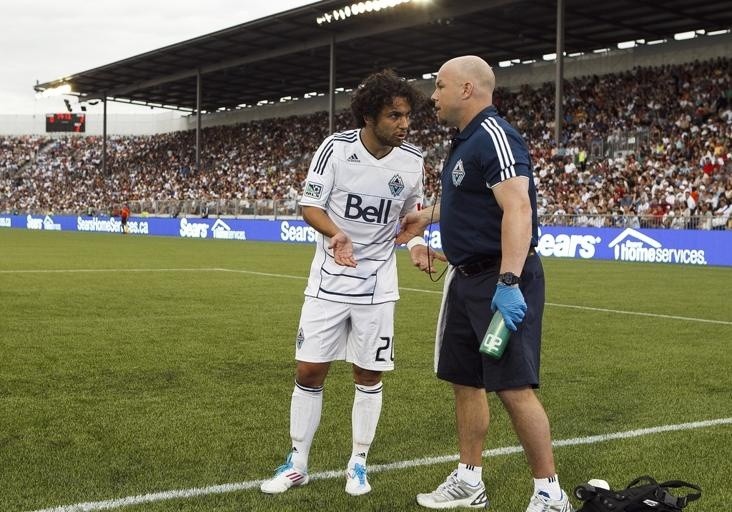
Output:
[454,263,492,277]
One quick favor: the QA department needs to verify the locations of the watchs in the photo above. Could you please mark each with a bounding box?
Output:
[494,272,521,286]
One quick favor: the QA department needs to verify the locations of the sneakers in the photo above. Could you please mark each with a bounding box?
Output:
[345,463,372,496]
[526,488,573,512]
[416,469,490,509]
[261,462,309,495]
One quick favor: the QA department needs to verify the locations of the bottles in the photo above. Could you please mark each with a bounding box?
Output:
[479,310,511,360]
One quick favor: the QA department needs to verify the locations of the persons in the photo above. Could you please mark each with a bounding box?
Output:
[492,58,732,230]
[0,108,359,234]
[393,55,575,512]
[401,96,457,210]
[257,67,446,498]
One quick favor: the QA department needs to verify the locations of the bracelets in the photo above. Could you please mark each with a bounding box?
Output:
[407,235,428,254]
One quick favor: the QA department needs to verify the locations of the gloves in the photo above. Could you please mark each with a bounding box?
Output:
[490,283,528,332]
